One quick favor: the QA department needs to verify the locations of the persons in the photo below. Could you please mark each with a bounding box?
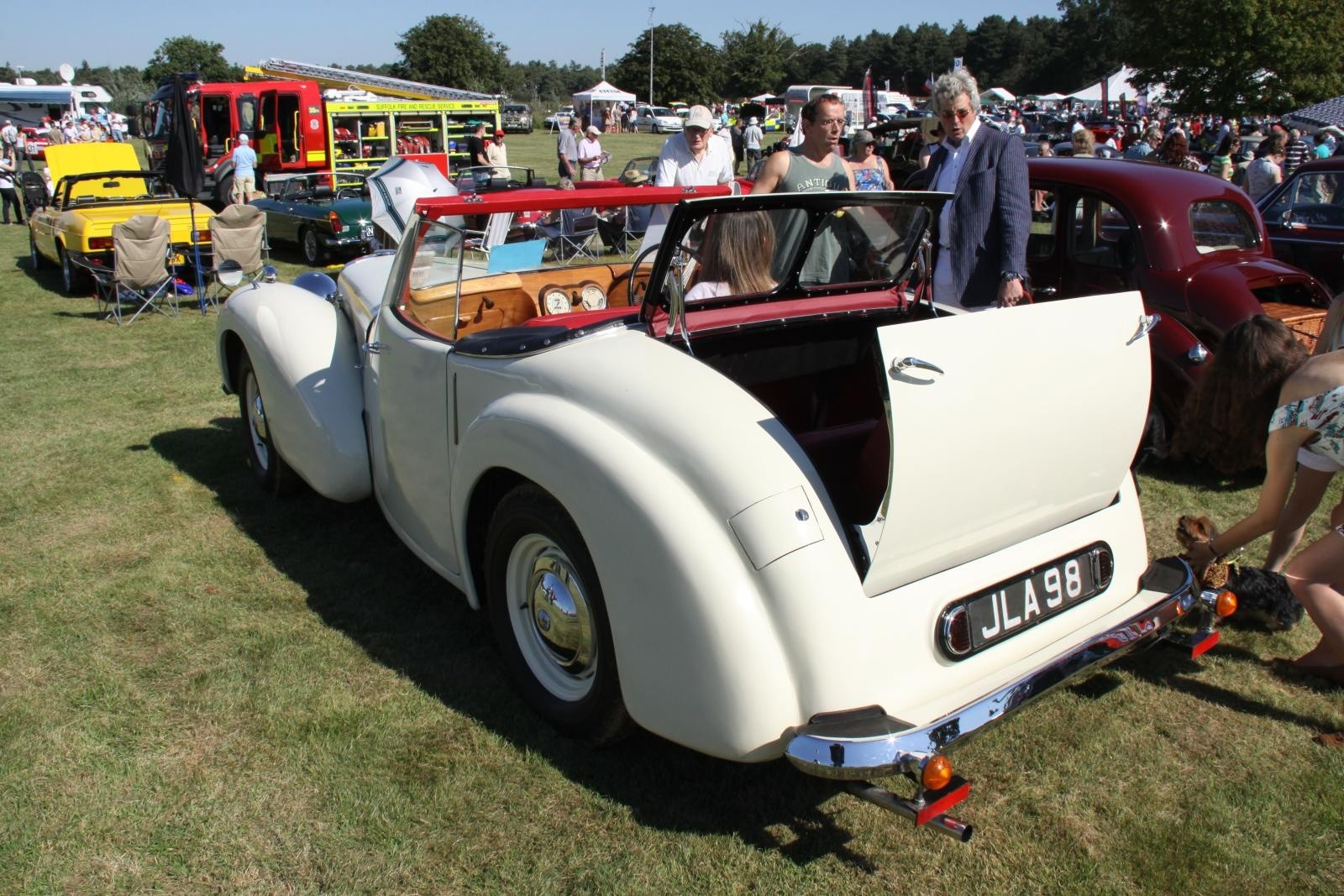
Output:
[470,123,494,185]
[558,117,582,181]
[867,102,1344,234]
[655,105,735,186]
[684,211,781,302]
[571,101,638,138]
[903,68,1032,313]
[486,130,512,187]
[750,94,856,194]
[847,129,894,192]
[1186,314,1344,676]
[0,108,125,224]
[670,97,794,176]
[577,126,604,181]
[230,133,257,205]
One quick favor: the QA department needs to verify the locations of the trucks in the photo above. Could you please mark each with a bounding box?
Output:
[0,78,129,137]
[127,79,504,213]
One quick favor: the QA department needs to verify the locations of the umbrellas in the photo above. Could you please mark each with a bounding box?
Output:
[365,155,465,245]
[166,72,205,230]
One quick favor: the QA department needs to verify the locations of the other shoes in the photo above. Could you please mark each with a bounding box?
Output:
[1273,658,1344,683]
[1320,729,1344,748]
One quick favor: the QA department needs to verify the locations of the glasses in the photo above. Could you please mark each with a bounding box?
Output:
[809,119,847,127]
[939,107,973,119]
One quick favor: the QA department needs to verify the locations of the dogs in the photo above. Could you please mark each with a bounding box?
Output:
[1176,514,1305,634]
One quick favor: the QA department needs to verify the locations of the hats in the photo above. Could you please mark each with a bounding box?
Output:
[853,130,874,145]
[723,121,732,127]
[749,117,758,124]
[587,125,601,135]
[494,130,504,136]
[685,105,712,129]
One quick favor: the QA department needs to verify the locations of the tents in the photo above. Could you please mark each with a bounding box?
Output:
[980,87,1016,104]
[572,81,636,126]
[1037,63,1292,111]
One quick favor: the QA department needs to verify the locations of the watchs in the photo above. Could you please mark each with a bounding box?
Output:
[1001,270,1019,281]
[1209,536,1220,555]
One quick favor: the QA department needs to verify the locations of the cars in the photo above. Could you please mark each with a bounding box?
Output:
[1191,156,1344,284]
[1238,135,1268,153]
[1027,157,1336,468]
[543,105,576,130]
[1020,110,1141,157]
[20,127,55,161]
[636,106,684,135]
[628,101,648,106]
[668,102,723,132]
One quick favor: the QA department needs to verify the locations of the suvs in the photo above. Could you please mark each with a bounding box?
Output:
[501,104,534,134]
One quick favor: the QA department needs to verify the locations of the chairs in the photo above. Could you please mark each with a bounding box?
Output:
[76,195,95,204]
[153,192,171,199]
[313,184,332,200]
[362,181,370,197]
[72,213,179,326]
[520,305,641,329]
[180,203,272,314]
[463,212,514,260]
[599,203,666,264]
[534,206,599,266]
[527,178,546,188]
[487,178,506,188]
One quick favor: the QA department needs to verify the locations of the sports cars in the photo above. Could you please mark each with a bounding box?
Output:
[246,173,378,267]
[21,142,218,296]
[214,181,1203,782]
